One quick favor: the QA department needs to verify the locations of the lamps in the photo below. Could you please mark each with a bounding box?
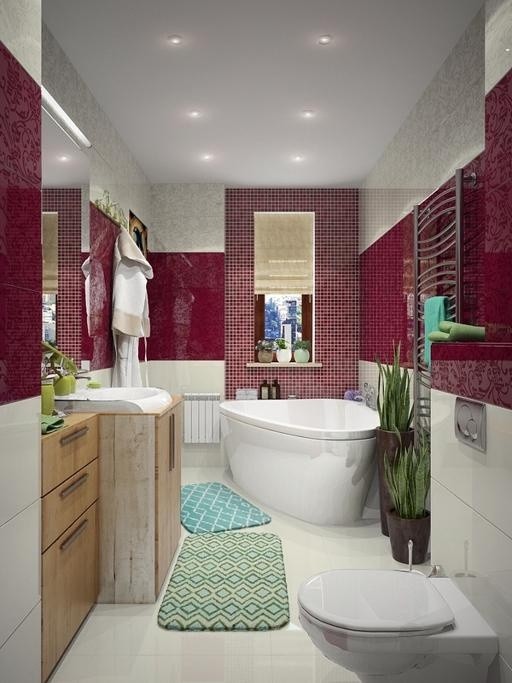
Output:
[40,85,92,148]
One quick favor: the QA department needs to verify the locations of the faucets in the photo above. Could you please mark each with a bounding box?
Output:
[64,369,92,381]
[353,382,378,411]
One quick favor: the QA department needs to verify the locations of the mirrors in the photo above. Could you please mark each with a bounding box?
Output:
[41,104,90,385]
[254,212,316,362]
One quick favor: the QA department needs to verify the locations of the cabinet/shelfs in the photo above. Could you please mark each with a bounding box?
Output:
[41,414,100,682]
[97,398,184,603]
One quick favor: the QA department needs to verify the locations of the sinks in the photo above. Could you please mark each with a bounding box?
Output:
[54,387,174,413]
[218,399,382,525]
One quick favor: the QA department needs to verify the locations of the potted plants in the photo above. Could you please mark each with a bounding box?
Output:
[376,340,414,536]
[275,339,292,363]
[255,341,274,363]
[292,341,310,363]
[384,433,431,564]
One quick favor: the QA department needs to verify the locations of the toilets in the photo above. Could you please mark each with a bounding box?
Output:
[297,568,499,682]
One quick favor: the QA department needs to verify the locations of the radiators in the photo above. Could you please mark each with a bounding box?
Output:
[184,393,221,445]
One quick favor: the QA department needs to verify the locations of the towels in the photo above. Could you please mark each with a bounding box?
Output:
[424,296,451,365]
[429,322,485,341]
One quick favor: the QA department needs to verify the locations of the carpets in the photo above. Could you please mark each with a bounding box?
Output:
[178,481,271,532]
[157,530,290,630]
